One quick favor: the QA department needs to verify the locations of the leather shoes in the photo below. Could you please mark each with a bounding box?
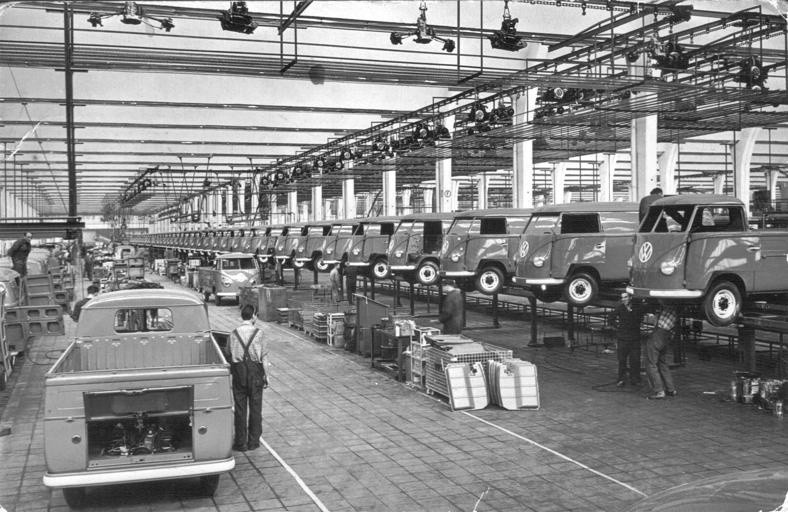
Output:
[664,391,677,397]
[616,379,624,387]
[248,445,261,450]
[648,392,666,400]
[231,443,249,453]
[631,380,645,387]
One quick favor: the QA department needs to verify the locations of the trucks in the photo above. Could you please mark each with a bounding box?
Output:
[194,252,260,306]
[38,287,259,510]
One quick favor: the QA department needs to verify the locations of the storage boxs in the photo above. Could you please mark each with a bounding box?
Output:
[426,341,514,406]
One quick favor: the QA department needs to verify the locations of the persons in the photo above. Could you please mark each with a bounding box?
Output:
[69,285,99,323]
[640,188,685,232]
[430,280,465,334]
[223,303,271,452]
[606,291,645,388]
[62,246,97,281]
[343,262,359,303]
[93,279,106,295]
[177,261,186,287]
[643,298,676,400]
[7,232,34,277]
[329,263,342,303]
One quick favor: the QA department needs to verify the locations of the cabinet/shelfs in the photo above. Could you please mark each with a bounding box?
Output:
[276,293,439,405]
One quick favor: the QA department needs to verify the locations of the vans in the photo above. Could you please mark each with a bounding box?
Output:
[272,210,457,288]
[511,199,715,309]
[625,193,788,328]
[114,223,285,263]
[437,207,536,295]
